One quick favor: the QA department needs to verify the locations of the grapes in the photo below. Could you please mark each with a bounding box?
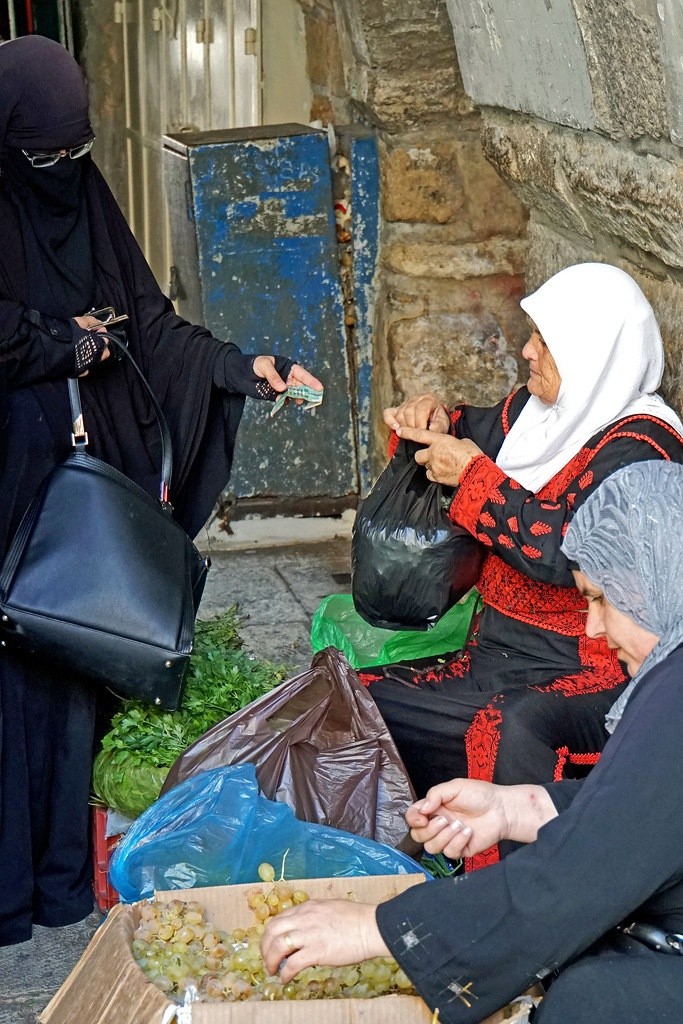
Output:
[132,863,412,1003]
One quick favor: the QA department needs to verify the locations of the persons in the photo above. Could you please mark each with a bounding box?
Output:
[352,262,683,877]
[0,35,324,946]
[261,461,683,1024]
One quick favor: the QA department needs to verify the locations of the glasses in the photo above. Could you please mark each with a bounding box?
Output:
[21,136,96,168]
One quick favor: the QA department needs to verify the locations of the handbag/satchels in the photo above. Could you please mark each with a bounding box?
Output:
[109,645,436,901]
[349,438,455,632]
[1,332,210,713]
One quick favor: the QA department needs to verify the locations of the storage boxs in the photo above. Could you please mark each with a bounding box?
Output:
[33,872,545,1024]
[91,805,129,912]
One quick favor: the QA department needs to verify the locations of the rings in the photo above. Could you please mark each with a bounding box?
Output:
[284,932,296,953]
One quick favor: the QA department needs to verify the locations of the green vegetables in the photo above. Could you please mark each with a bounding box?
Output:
[91,602,290,817]
